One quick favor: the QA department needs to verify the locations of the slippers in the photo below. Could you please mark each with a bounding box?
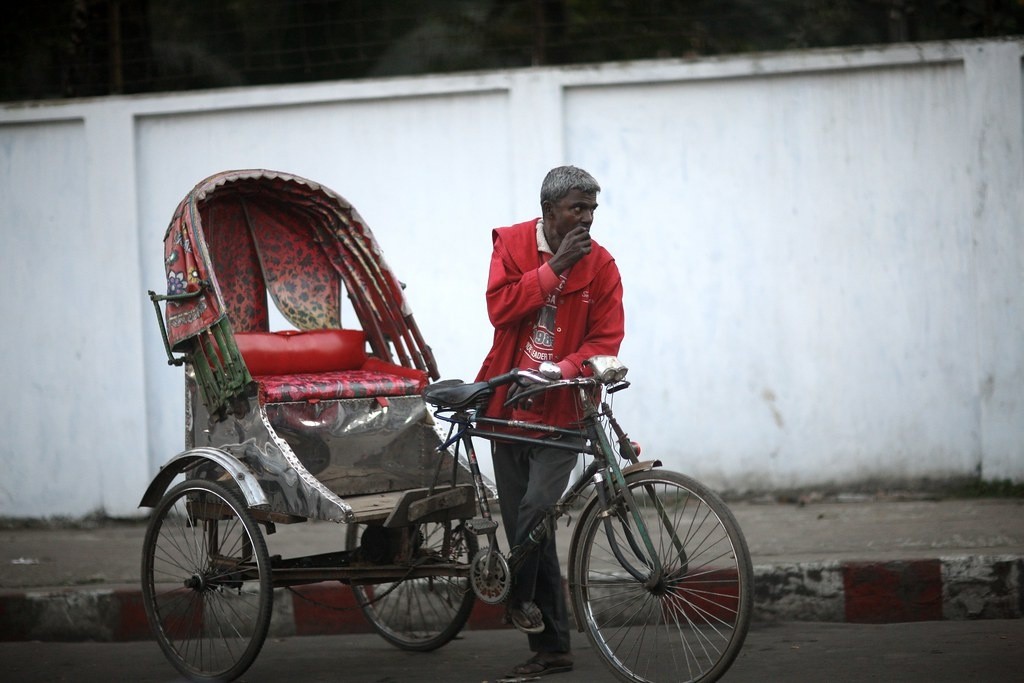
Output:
[505,600,545,633]
[504,656,573,677]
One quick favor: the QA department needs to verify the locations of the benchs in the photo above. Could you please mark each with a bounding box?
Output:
[200,328,428,404]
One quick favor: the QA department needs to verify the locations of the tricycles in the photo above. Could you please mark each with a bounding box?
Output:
[134,166,756,683]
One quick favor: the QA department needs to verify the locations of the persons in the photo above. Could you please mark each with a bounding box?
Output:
[475,166,624,677]
